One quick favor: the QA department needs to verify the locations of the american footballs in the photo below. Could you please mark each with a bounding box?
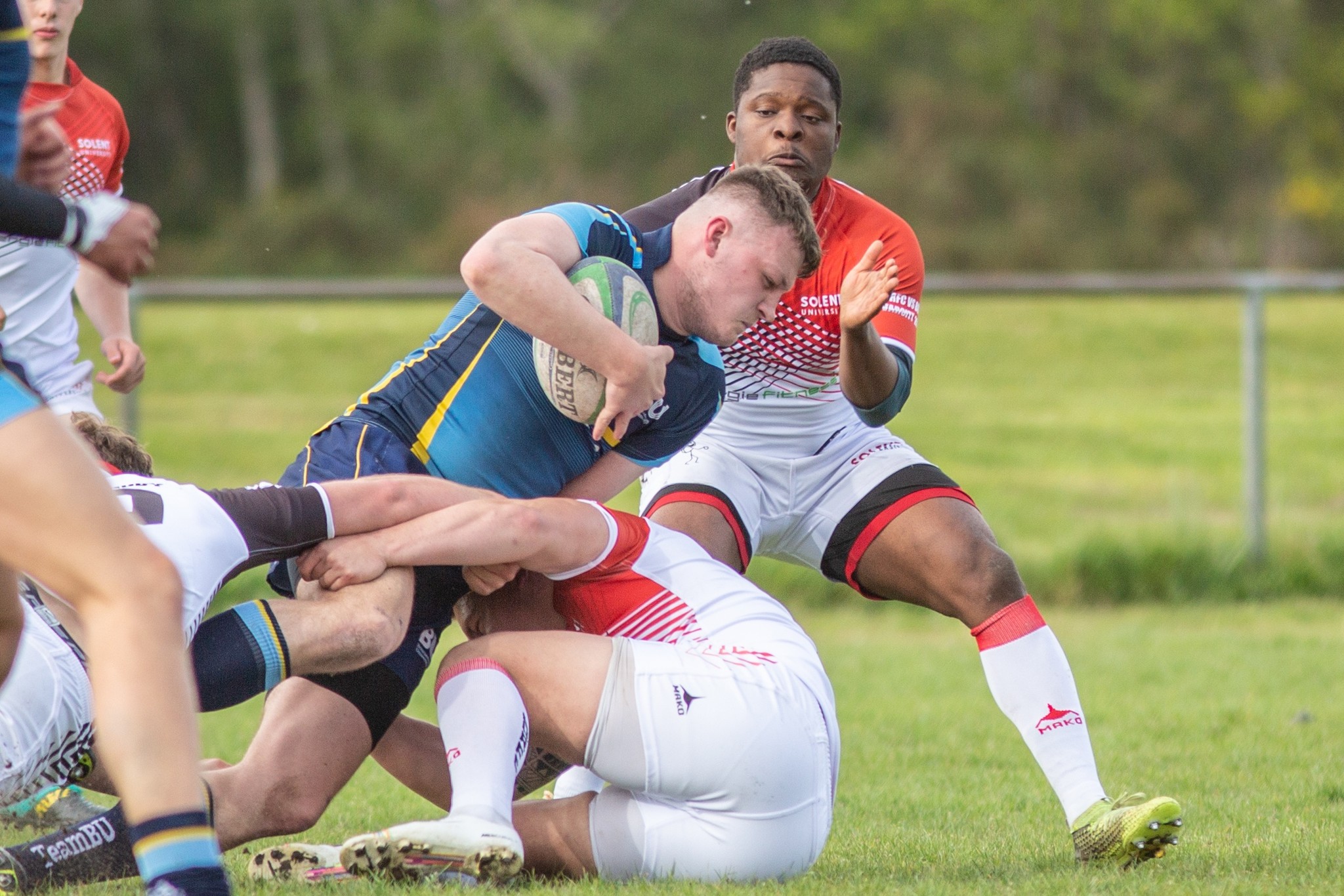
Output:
[530,253,657,425]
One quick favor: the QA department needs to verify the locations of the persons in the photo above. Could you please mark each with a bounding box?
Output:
[0,0,841,896]
[619,34,1183,870]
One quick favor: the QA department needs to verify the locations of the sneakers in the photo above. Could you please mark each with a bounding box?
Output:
[1065,792,1184,869]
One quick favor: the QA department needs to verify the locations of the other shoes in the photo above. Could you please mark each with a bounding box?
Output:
[0,847,28,896]
[13,788,110,834]
[249,844,340,883]
[338,812,524,883]
